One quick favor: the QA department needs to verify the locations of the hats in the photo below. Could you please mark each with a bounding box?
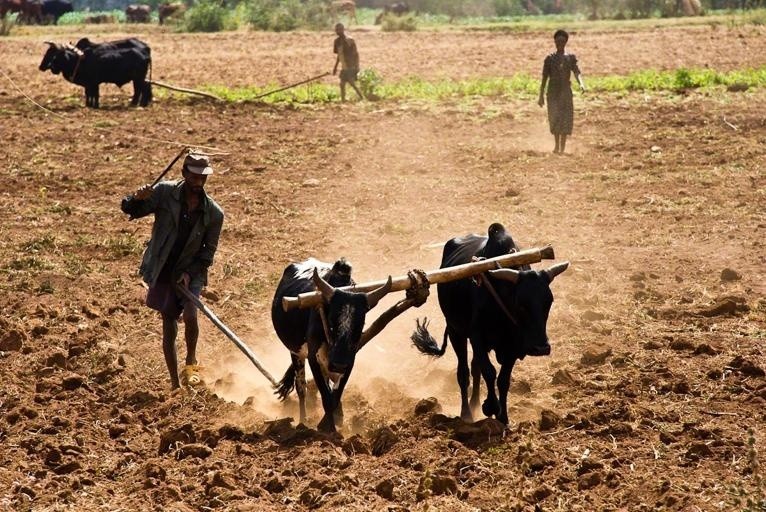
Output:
[183,150,214,176]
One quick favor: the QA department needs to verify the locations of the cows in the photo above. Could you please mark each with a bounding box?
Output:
[269,256,393,433]
[409,222,570,427]
[38,37,153,109]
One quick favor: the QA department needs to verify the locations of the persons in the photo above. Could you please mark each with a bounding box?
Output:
[332,23,363,103]
[121,150,224,388]
[538,30,586,155]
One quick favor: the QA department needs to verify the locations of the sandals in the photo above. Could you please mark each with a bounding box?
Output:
[169,360,201,397]
